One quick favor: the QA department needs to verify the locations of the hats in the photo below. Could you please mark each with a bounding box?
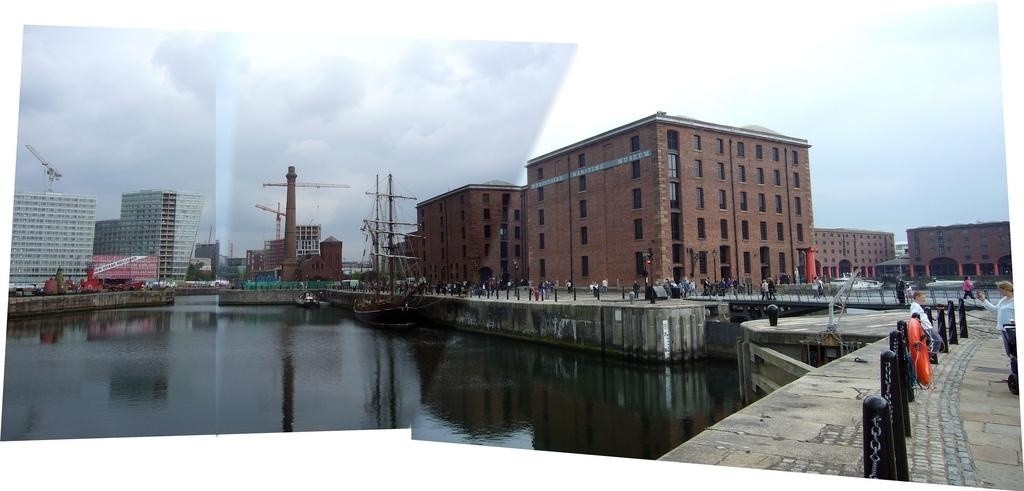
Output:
[995,281,1013,291]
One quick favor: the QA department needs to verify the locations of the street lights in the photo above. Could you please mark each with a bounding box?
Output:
[646,248,656,305]
[712,250,718,283]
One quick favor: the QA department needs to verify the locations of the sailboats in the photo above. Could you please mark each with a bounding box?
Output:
[353,172,430,331]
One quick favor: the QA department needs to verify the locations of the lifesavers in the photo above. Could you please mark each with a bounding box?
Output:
[908,317,932,386]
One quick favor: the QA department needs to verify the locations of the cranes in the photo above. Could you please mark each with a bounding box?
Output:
[254,202,287,268]
[262,182,353,191]
[23,143,63,193]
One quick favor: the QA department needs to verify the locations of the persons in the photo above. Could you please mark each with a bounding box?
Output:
[633,281,640,298]
[590,280,608,297]
[976,280,1017,384]
[962,276,976,300]
[904,282,913,304]
[761,278,776,301]
[538,280,554,299]
[567,280,571,293]
[817,278,826,298]
[810,277,820,300]
[438,281,494,297]
[908,311,930,347]
[500,277,529,290]
[664,278,698,297]
[703,277,737,296]
[895,275,906,305]
[909,293,945,362]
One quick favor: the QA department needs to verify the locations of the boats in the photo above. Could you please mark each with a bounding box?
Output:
[822,273,886,293]
[295,292,319,311]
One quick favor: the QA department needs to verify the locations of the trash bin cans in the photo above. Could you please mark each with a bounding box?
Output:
[671,288,680,298]
